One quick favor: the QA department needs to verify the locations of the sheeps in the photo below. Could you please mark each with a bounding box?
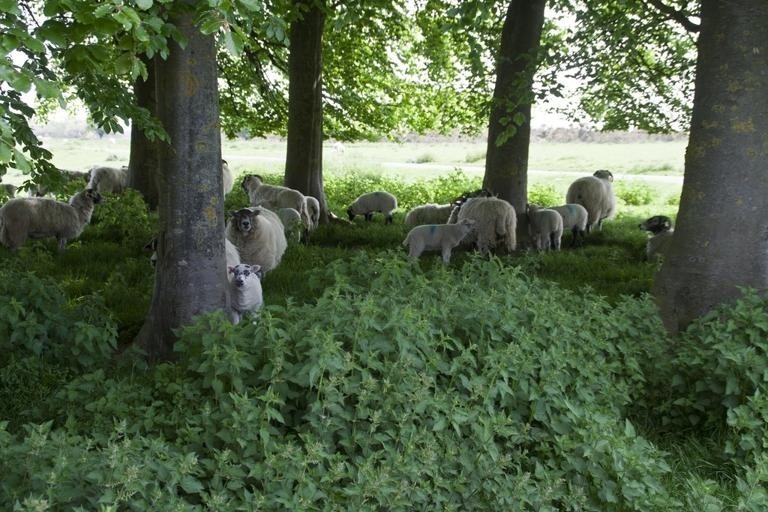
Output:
[566,170,616,235]
[542,203,589,245]
[403,196,517,270]
[347,191,396,225]
[224,174,320,283]
[144,237,241,310]
[0,189,103,255]
[227,263,263,327]
[222,159,233,200]
[526,203,563,251]
[84,166,129,194]
[637,215,675,264]
